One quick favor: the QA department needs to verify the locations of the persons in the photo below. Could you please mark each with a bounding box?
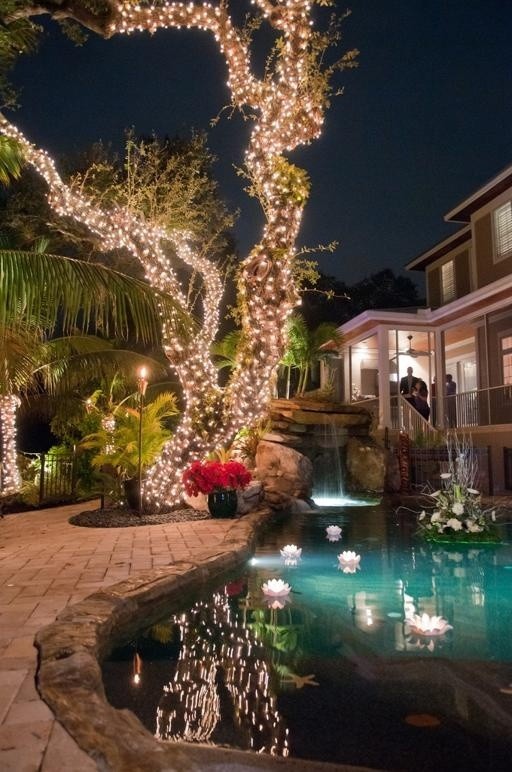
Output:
[431,377,435,428]
[410,377,430,428]
[398,367,420,431]
[445,374,457,429]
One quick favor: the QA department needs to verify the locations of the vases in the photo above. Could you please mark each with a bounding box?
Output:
[208,488,236,517]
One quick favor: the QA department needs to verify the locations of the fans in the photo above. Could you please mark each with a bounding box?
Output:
[394,336,431,358]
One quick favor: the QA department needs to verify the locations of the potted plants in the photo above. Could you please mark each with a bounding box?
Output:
[80,392,180,508]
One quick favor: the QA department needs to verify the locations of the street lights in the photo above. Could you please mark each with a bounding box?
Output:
[137,365,148,513]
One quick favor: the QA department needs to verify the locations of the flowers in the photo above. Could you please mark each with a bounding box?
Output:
[183,447,252,496]
